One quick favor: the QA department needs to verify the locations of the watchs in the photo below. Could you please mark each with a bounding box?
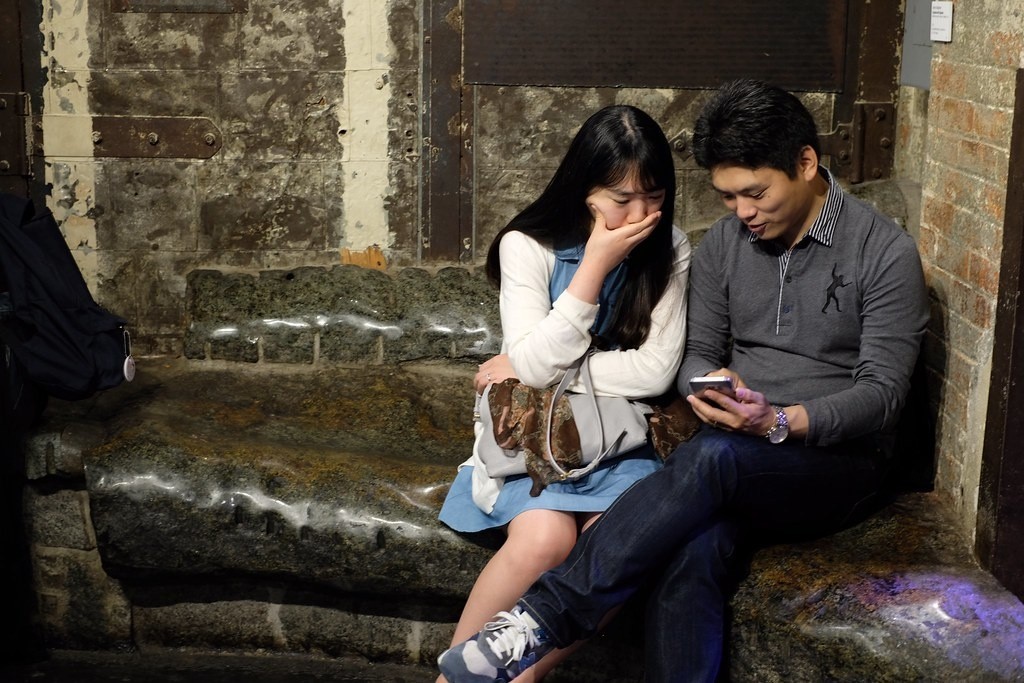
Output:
[765,405,790,445]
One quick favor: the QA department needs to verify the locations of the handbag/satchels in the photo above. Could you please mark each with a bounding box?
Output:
[473,347,657,499]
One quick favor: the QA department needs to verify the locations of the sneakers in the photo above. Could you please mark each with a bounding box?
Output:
[437,606,555,683]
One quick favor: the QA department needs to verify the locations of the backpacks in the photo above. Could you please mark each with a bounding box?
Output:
[0,192,136,410]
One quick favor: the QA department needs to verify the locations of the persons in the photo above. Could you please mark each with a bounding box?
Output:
[439,104,692,682]
[0,244,32,683]
[437,80,931,683]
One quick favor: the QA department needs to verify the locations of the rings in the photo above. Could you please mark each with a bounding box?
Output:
[487,374,491,382]
[712,419,717,427]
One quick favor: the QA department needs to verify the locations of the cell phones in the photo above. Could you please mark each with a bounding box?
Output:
[690,376,739,411]
